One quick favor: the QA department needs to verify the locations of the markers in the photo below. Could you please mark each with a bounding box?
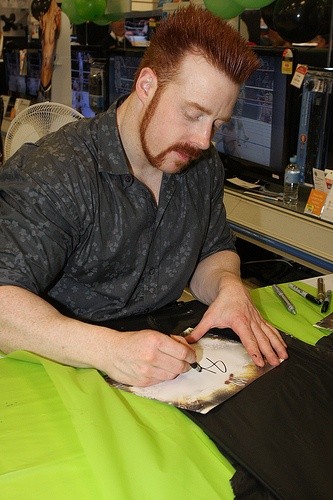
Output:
[288,282,321,305]
[317,278,325,302]
[320,289,331,313]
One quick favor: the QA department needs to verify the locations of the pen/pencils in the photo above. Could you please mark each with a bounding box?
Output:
[147,315,203,372]
[272,283,297,315]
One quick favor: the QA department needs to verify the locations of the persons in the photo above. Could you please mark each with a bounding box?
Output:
[106,20,132,45]
[316,34,330,48]
[0,6,288,388]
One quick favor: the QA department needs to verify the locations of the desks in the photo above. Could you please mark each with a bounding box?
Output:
[0,179,333,499]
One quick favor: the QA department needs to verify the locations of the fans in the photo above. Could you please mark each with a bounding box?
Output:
[4,101,84,161]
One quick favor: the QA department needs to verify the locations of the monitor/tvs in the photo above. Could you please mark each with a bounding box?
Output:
[208,53,290,191]
[104,47,147,111]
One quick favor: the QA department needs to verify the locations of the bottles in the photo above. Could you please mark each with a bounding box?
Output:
[284,158,300,207]
